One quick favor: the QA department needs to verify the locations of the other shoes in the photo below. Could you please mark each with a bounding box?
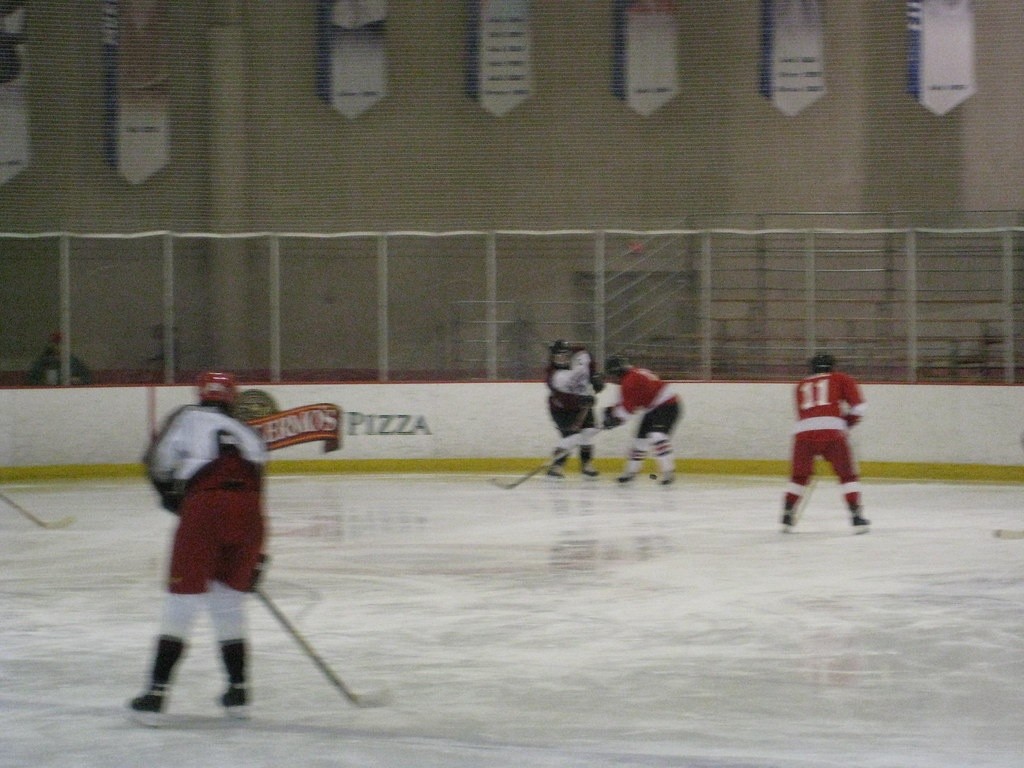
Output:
[655,470,677,490]
[618,473,637,485]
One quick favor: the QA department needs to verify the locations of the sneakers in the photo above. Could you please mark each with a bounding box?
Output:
[546,464,569,482]
[850,504,873,534]
[780,514,795,533]
[125,690,165,727]
[581,458,603,481]
[221,682,251,720]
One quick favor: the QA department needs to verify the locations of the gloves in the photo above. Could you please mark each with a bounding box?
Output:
[576,394,595,410]
[162,491,178,515]
[602,406,621,428]
[590,372,605,392]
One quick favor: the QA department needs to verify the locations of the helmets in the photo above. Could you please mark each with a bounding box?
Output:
[549,338,573,368]
[605,355,630,378]
[198,371,236,408]
[809,352,837,374]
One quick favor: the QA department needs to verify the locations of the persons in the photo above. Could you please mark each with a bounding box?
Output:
[126,367,273,724]
[782,352,869,534]
[600,355,681,491]
[29,329,91,389]
[545,339,608,484]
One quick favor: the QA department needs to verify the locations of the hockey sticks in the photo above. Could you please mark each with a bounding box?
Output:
[1,492,76,531]
[488,432,600,490]
[990,528,1023,542]
[256,583,393,710]
